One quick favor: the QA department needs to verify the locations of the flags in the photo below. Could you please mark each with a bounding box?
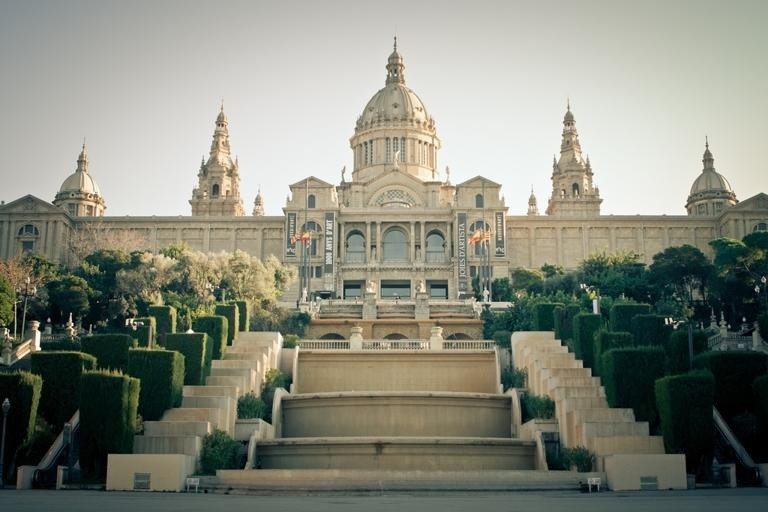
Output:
[467,227,491,248]
[289,228,312,249]
